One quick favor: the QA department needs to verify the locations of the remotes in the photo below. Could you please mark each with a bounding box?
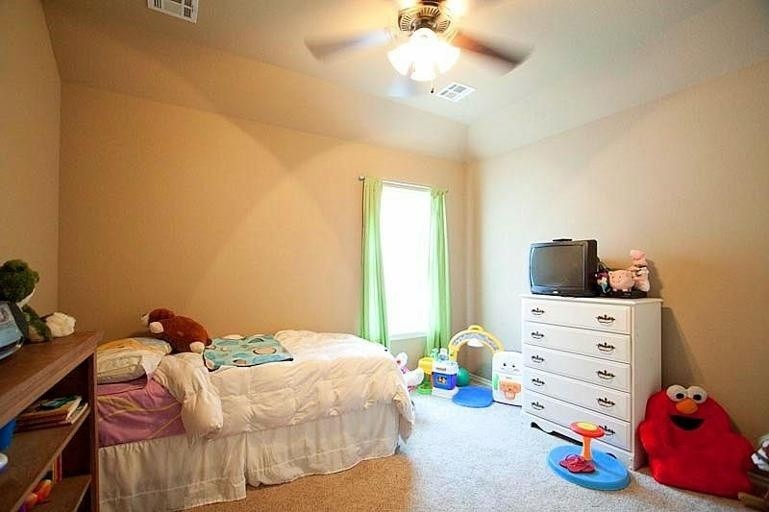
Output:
[553,238,571,242]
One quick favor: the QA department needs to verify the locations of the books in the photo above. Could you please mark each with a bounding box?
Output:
[51,454,63,484]
[16,395,89,432]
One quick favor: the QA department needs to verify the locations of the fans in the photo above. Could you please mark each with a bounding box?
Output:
[303,0,531,98]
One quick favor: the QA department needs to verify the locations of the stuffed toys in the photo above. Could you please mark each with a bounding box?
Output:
[593,249,651,293]
[140,308,212,353]
[638,383,756,500]
[0,259,76,343]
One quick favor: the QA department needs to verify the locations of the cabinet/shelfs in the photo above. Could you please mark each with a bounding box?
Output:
[519,294,664,471]
[0,331,98,511]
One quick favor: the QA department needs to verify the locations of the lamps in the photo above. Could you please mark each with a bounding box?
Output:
[386,3,459,82]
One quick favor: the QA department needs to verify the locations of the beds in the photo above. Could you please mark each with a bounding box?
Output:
[39,312,415,512]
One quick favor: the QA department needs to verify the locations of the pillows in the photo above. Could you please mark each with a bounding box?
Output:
[94,337,172,384]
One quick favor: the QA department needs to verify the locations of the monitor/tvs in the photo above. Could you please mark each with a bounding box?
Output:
[527,240,598,298]
